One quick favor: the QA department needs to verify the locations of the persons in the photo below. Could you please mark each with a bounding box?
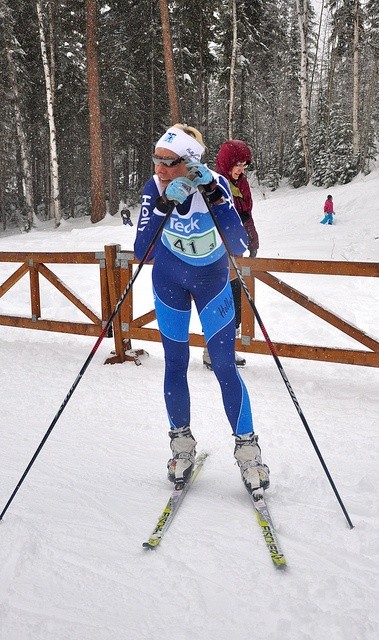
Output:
[134,121,269,494]
[198,141,259,369]
[120,208,133,227]
[320,195,335,226]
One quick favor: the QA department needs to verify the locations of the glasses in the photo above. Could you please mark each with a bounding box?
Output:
[234,164,249,169]
[150,154,184,168]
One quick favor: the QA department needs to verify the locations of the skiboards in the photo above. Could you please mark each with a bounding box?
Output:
[142,451,288,572]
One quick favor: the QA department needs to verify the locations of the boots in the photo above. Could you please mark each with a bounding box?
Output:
[231,432,270,493]
[203,330,213,367]
[234,326,246,366]
[167,426,197,483]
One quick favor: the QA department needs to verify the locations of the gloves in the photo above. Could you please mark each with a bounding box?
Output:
[184,161,224,203]
[324,212,328,216]
[332,211,335,215]
[153,177,194,214]
[248,248,257,258]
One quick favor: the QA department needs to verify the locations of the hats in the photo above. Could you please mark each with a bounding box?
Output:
[327,195,332,199]
